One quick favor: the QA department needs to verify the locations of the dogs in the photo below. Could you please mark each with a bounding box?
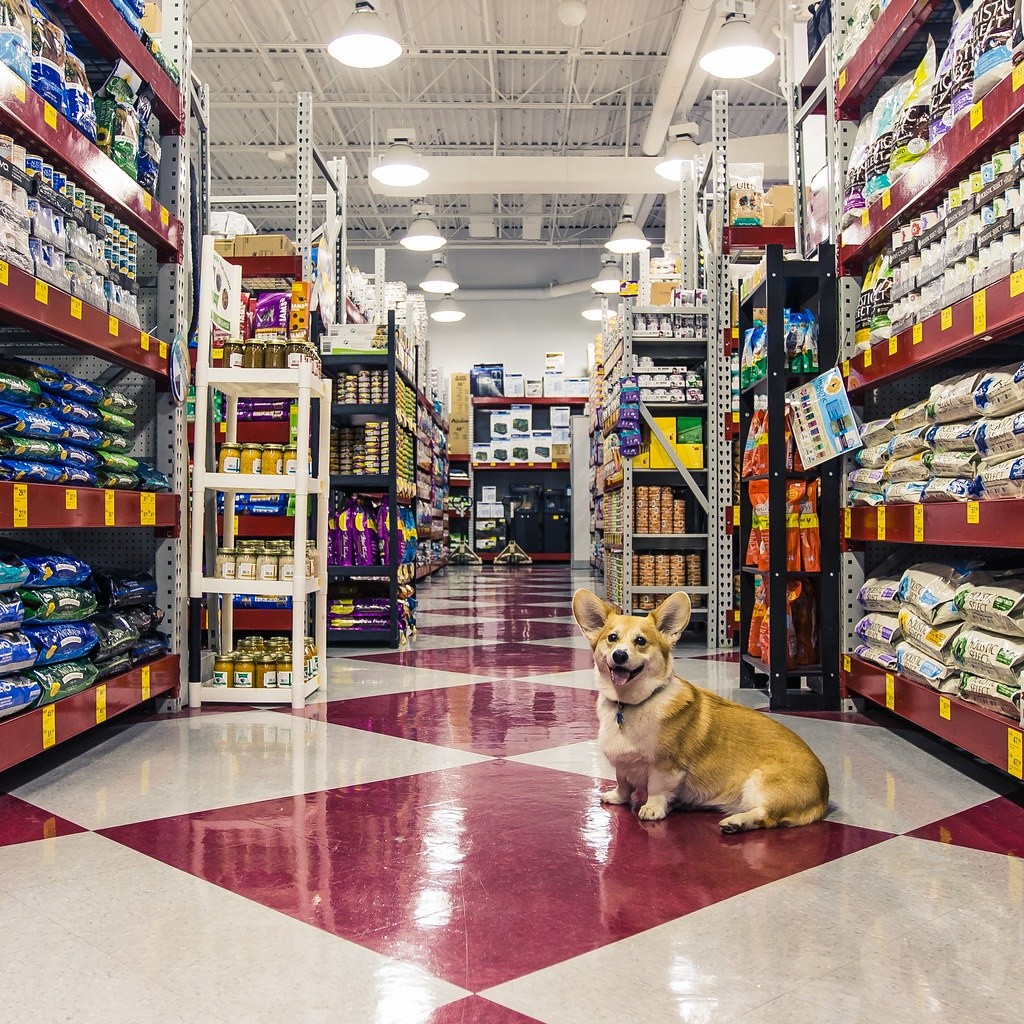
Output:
[572,588,830,833]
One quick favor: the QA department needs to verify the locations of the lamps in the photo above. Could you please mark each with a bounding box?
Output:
[395,201,447,254]
[655,121,705,181]
[326,0,403,69]
[588,251,626,296]
[371,126,428,188]
[420,252,461,294]
[579,289,616,324]
[429,291,468,324]
[604,204,652,254]
[699,0,777,79]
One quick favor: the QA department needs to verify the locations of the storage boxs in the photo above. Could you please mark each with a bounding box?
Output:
[776,210,794,226]
[650,415,676,443]
[214,239,235,256]
[763,203,775,225]
[646,444,677,469]
[650,281,679,304]
[678,416,703,443]
[632,451,649,468]
[234,234,296,255]
[677,443,704,469]
[768,182,813,226]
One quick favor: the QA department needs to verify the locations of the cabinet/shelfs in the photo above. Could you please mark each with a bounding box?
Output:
[0,0,1024,790]
